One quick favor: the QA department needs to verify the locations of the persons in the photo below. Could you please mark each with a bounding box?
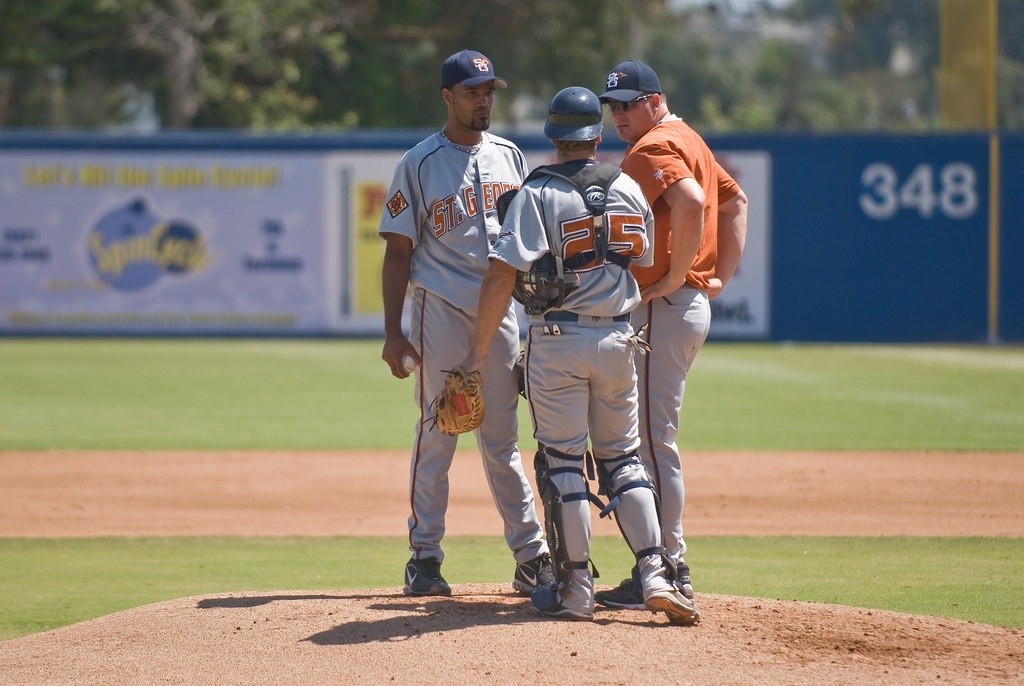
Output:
[445,88,701,625]
[594,60,748,610]
[378,50,556,596]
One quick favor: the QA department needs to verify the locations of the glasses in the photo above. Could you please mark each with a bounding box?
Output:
[607,94,652,112]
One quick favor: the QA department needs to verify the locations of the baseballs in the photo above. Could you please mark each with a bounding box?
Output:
[402,355,417,374]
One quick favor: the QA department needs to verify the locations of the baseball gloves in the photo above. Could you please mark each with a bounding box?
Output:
[433,365,486,435]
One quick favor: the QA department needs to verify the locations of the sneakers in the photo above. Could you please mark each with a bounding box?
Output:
[646,589,700,626]
[594,579,647,610]
[513,552,556,597]
[675,564,694,599]
[531,583,593,621]
[403,558,451,595]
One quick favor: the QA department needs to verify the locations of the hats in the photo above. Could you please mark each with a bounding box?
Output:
[439,48,510,89]
[598,59,662,101]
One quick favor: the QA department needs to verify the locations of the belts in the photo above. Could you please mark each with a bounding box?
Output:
[544,310,630,322]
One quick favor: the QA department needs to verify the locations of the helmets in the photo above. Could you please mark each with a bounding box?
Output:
[544,86,602,140]
